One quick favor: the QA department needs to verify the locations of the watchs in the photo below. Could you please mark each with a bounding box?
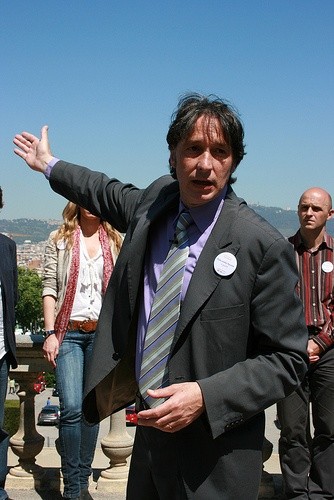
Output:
[42,330,55,339]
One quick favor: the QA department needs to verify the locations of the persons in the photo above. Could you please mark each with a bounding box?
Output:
[0,185,18,500]
[42,200,125,500]
[13,92,310,500]
[47,397,50,405]
[273,187,334,500]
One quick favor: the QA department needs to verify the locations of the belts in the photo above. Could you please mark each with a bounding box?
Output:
[66,319,97,333]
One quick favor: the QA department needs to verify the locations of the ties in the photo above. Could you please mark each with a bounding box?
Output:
[137,213,192,409]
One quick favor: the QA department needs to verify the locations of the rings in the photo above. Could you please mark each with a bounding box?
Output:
[167,423,173,431]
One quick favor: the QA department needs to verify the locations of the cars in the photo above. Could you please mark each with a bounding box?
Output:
[125,405,137,426]
[33,374,47,395]
[36,405,61,426]
[51,383,59,396]
